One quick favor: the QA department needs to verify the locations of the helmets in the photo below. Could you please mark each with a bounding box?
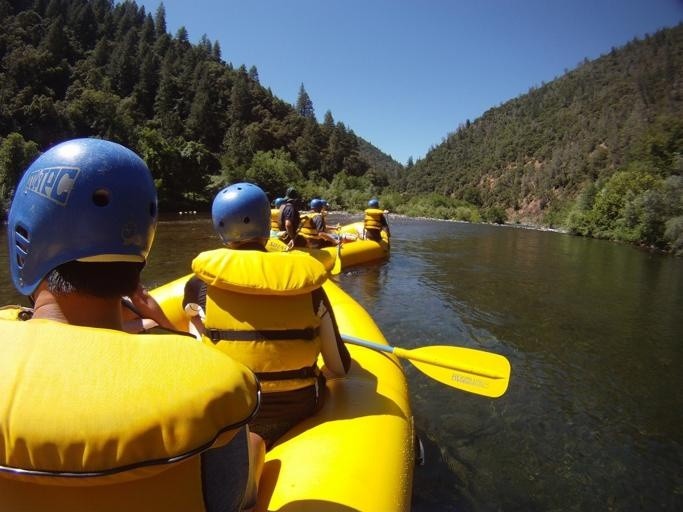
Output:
[368,199,379,208]
[7,137,159,296]
[273,186,327,208]
[211,182,272,244]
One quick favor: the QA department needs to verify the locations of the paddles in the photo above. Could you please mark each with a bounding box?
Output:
[332,229,341,274]
[341,338,511,398]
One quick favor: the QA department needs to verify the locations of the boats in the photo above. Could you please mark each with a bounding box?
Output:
[122,274,414,511]
[266,223,390,269]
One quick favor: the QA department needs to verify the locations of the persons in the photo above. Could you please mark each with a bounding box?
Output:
[319,199,342,235]
[270,198,283,239]
[280,187,313,251]
[299,198,340,246]
[357,199,390,240]
[0,138,266,512]
[177,182,349,452]
